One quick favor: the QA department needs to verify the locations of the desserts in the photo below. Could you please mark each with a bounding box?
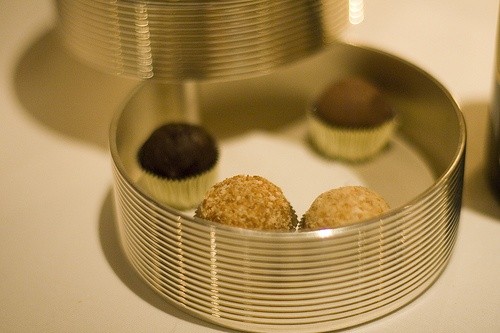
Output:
[132,120,218,210]
[196,174,298,235]
[304,74,397,164]
[300,183,390,233]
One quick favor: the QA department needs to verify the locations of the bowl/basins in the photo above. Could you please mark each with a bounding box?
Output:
[106,44,468,333]
